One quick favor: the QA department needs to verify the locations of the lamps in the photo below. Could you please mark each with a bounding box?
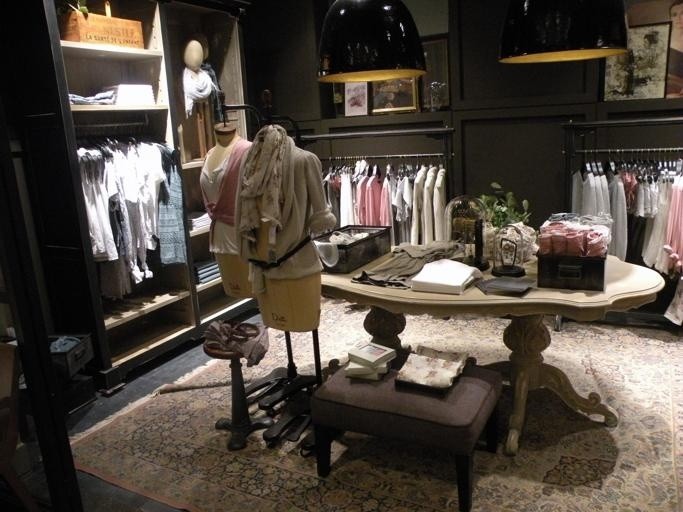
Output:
[489,0,633,71]
[312,2,425,88]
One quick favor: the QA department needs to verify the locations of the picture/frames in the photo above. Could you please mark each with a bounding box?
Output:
[367,76,418,116]
[601,21,673,103]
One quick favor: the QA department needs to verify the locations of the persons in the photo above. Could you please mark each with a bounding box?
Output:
[372,79,413,109]
[664,0,683,99]
[198,115,253,300]
[233,125,338,333]
[197,35,234,123]
[179,40,218,159]
[632,31,658,82]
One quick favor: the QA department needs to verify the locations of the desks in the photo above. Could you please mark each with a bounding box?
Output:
[314,241,666,457]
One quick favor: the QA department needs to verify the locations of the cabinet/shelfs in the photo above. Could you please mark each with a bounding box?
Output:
[158,4,260,336]
[27,0,200,400]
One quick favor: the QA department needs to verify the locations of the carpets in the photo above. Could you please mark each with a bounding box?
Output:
[66,292,683,511]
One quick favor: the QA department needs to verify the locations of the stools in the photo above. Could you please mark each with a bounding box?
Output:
[309,349,508,512]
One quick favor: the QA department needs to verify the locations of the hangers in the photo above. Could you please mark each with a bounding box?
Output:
[566,142,680,186]
[321,152,446,181]
[74,128,149,161]
